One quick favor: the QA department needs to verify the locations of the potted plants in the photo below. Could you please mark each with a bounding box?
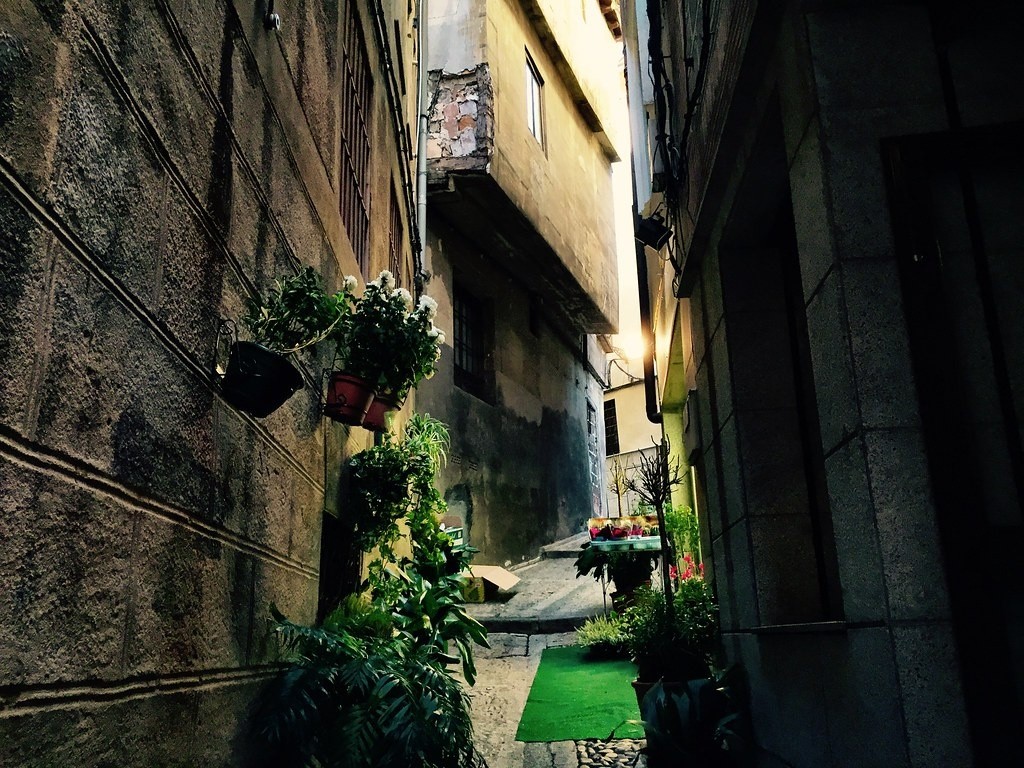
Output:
[221,264,332,418]
[574,610,629,658]
[606,453,653,541]
[625,433,724,768]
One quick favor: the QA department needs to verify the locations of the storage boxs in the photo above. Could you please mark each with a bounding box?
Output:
[440,515,463,547]
[455,565,521,604]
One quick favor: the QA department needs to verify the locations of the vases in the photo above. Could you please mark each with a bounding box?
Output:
[324,371,376,425]
[361,394,405,435]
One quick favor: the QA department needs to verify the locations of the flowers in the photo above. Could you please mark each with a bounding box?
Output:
[335,268,445,398]
